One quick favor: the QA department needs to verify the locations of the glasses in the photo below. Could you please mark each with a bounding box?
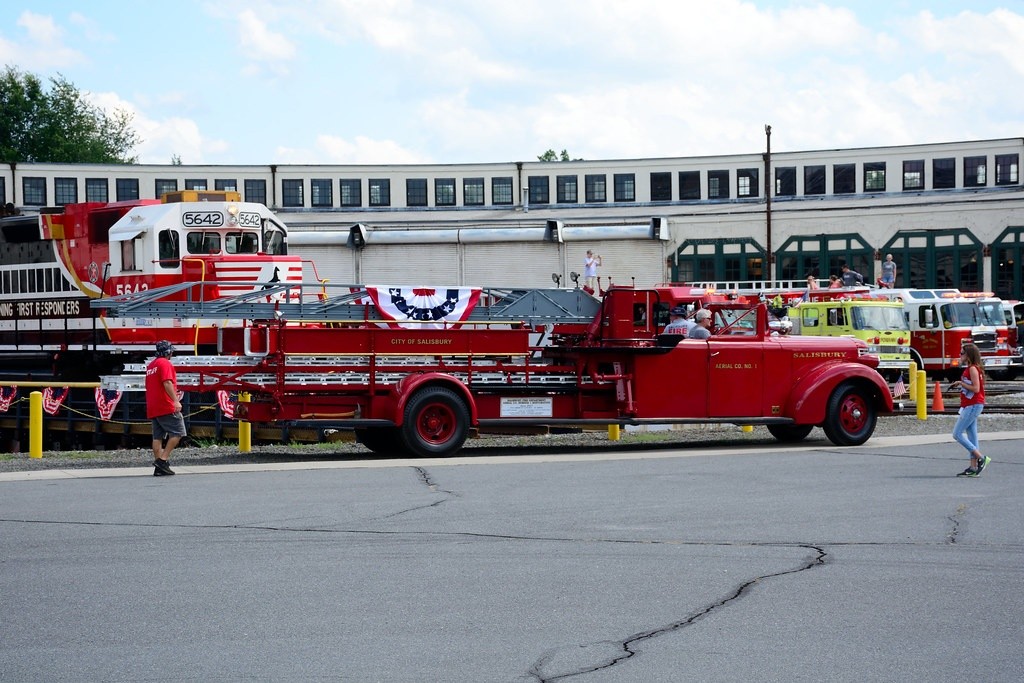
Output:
[703,318,711,320]
[841,267,845,271]
[961,352,967,355]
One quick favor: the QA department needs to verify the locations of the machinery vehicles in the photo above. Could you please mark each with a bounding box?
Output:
[88,257,892,446]
[652,289,910,384]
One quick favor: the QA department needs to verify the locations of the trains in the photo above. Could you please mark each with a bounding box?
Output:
[0,194,306,374]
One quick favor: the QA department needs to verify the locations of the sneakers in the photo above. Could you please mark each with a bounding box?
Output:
[975,455,991,475]
[153,458,175,476]
[956,467,980,477]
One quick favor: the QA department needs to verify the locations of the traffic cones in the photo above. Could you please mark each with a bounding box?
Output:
[930,381,945,413]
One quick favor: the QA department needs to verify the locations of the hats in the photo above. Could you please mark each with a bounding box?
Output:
[587,250,595,255]
[155,340,176,357]
[670,307,687,318]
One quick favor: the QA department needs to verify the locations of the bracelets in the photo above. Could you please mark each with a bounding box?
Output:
[960,381,964,385]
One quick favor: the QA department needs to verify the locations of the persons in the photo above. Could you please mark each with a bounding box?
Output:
[639,307,646,319]
[688,308,712,339]
[948,343,991,477]
[804,275,818,303]
[829,264,863,288]
[145,339,187,476]
[881,254,896,289]
[663,307,697,339]
[584,250,602,289]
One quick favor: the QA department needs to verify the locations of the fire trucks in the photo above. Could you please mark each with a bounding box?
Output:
[675,281,1024,380]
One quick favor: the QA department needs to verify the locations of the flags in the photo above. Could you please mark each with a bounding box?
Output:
[893,375,906,397]
